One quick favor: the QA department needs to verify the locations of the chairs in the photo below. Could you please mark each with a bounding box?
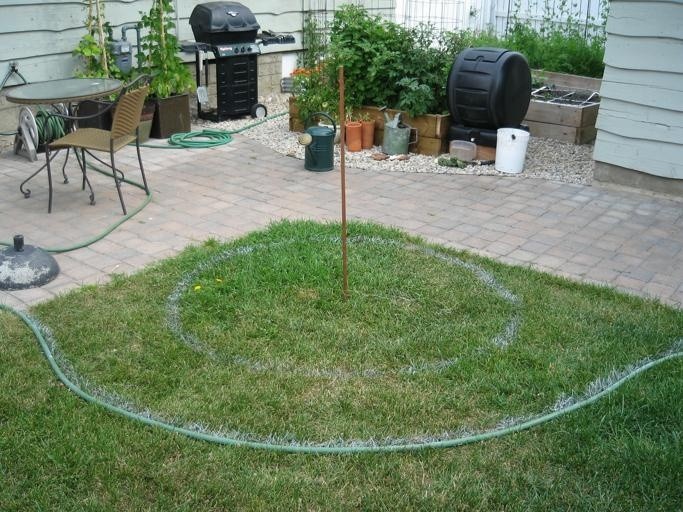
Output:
[42,72,153,215]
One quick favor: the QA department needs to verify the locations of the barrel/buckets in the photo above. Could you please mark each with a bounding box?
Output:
[446,46,532,145]
[494,127,530,175]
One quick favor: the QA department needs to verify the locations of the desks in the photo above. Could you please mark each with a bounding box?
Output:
[6,77,123,206]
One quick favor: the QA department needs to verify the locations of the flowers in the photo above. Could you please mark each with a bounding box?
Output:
[307,95,341,125]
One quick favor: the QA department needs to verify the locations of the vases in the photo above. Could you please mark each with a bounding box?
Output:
[317,120,341,144]
[346,122,362,152]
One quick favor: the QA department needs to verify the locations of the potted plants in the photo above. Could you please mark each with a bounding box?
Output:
[358,112,376,149]
